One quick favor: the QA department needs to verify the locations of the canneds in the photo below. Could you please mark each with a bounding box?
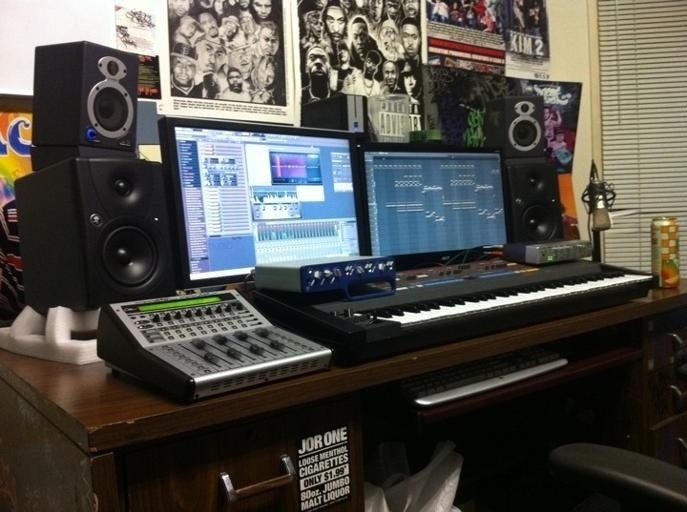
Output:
[651,218,679,288]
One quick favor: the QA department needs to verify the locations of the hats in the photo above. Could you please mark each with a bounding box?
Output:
[170,43,197,65]
[221,15,241,29]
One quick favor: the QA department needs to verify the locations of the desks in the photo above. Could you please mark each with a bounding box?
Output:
[0,273,687,512]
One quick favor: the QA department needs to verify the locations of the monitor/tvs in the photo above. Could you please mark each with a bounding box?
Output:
[157,116,364,291]
[357,142,513,271]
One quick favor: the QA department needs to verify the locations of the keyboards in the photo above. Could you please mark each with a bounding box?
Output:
[400,346,569,409]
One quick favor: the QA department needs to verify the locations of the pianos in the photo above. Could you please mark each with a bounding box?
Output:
[252,260,656,367]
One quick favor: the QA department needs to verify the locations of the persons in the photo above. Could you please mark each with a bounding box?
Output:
[424,0,541,34]
[297,0,421,127]
[168,0,280,103]
[542,104,568,160]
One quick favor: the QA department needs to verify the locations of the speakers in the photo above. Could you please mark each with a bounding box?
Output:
[507,162,564,244]
[13,157,178,315]
[487,96,545,167]
[30,41,138,172]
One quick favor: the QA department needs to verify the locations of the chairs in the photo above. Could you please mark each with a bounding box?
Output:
[534,438,687,510]
